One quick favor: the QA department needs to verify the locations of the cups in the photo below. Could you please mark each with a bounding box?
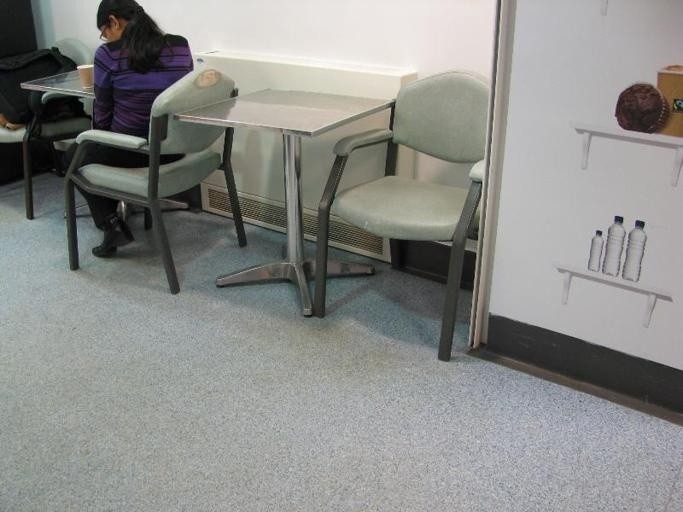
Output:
[77,64,94,92]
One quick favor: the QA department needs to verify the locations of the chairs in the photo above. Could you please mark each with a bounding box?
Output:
[63,65,247,294]
[2,112,94,220]
[314,54,492,361]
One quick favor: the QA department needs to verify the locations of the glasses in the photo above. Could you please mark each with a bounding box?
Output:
[99,27,108,41]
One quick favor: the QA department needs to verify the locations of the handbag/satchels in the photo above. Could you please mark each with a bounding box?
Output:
[0,45,80,126]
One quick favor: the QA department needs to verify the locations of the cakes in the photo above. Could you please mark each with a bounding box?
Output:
[614,83,670,133]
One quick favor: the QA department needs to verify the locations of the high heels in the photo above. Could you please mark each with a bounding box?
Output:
[93,219,133,257]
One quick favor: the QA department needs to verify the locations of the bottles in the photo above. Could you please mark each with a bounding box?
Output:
[589,215,647,281]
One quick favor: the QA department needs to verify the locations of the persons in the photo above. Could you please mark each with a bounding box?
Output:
[60,1,198,259]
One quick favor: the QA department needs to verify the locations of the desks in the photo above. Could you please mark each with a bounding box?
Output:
[174,85,395,318]
[19,65,98,101]
